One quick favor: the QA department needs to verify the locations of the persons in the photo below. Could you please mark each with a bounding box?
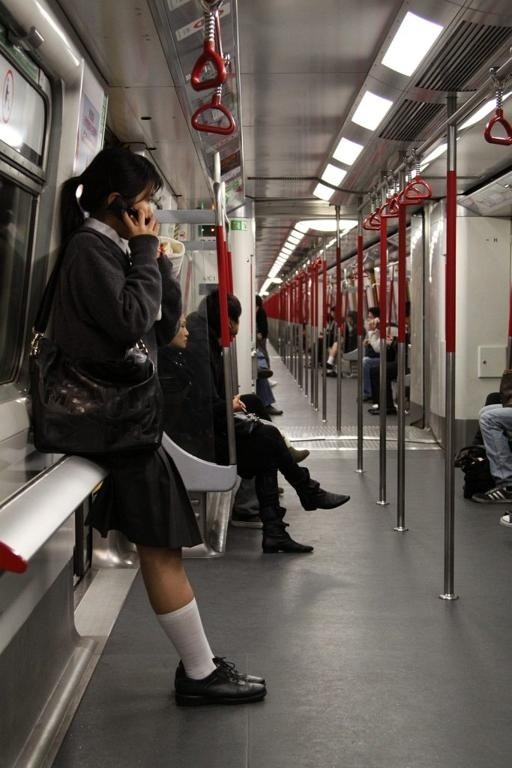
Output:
[312,302,413,417]
[159,306,351,554]
[469,348,512,505]
[186,286,294,528]
[37,147,272,706]
[235,290,310,464]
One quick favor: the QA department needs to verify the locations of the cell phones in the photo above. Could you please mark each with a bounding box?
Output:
[367,318,381,325]
[107,197,151,227]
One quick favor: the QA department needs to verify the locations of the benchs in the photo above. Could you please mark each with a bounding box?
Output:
[334,340,411,414]
[160,417,242,559]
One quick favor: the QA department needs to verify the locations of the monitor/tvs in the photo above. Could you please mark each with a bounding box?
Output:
[195,223,216,241]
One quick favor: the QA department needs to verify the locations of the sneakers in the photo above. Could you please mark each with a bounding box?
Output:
[498,509,512,528]
[470,482,512,506]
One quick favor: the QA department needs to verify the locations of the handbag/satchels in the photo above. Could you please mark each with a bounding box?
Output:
[453,443,495,500]
[23,227,170,456]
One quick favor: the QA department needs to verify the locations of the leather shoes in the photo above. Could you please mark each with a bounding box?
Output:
[368,406,396,416]
[168,656,269,707]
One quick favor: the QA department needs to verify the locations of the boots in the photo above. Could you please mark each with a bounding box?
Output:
[287,443,312,463]
[258,504,316,556]
[285,468,352,511]
